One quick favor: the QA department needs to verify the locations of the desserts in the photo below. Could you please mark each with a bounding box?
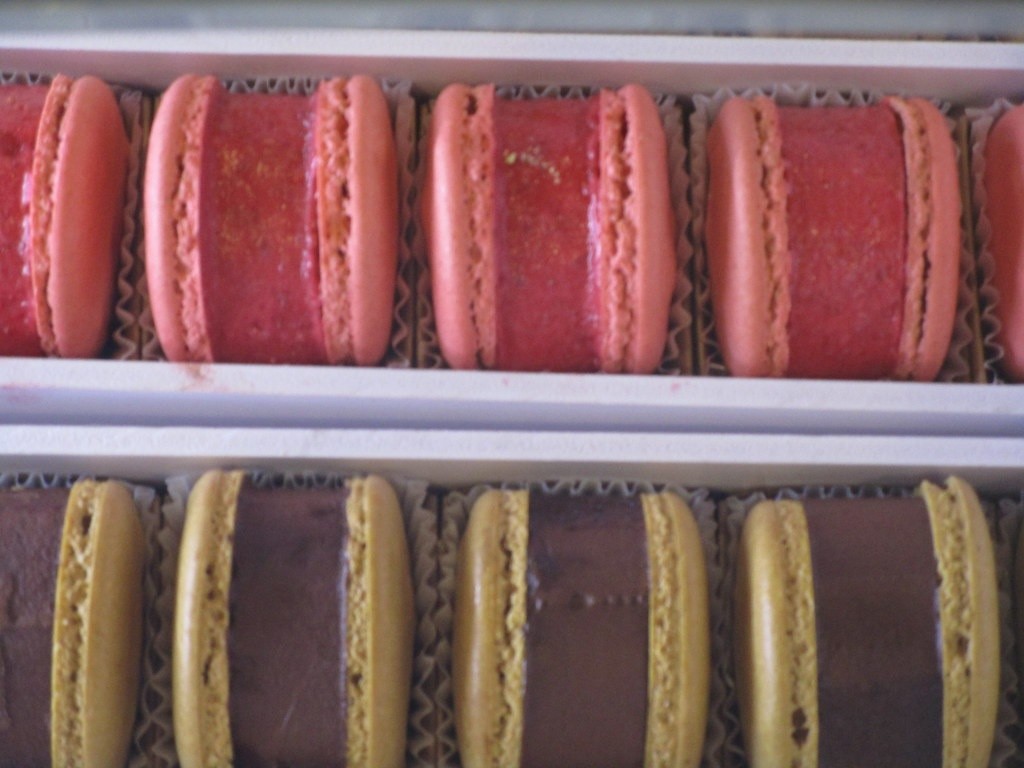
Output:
[0,71,1024,768]
